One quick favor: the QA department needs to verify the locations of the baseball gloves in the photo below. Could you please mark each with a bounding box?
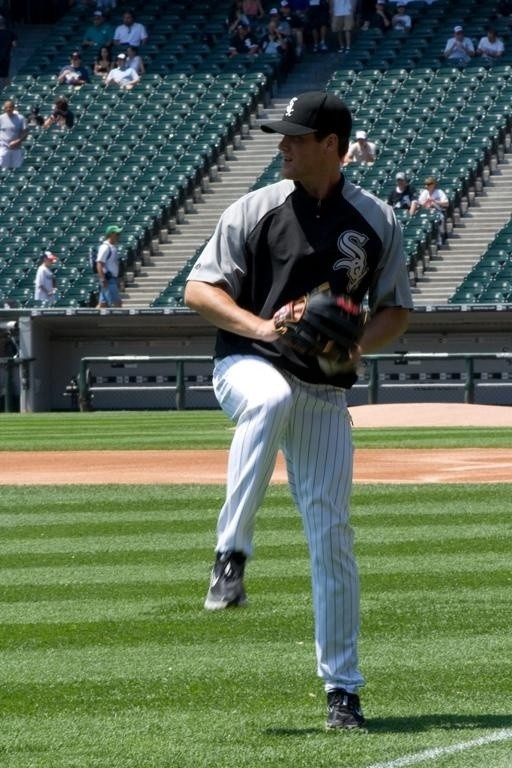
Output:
[274,292,364,361]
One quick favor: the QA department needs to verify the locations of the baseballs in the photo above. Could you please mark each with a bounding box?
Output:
[318,357,341,378]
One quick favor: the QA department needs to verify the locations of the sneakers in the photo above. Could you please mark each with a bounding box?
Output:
[203,550,247,611]
[313,42,352,55]
[324,689,367,732]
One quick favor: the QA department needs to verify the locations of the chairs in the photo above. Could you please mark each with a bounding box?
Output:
[2,0,316,312]
[324,0,512,312]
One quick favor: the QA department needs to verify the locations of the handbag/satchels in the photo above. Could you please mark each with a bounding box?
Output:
[88,243,111,273]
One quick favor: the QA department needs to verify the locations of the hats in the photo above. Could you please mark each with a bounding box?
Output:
[68,51,81,59]
[258,90,352,137]
[115,53,127,62]
[355,130,368,140]
[453,25,465,35]
[395,2,406,8]
[395,171,407,181]
[236,0,290,29]
[43,250,57,260]
[91,11,103,21]
[376,0,386,6]
[105,225,124,238]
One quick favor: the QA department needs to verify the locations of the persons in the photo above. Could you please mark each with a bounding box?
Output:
[388,172,417,208]
[94,224,124,308]
[345,131,375,163]
[411,177,449,247]
[184,91,416,728]
[1,0,154,176]
[34,250,55,308]
[476,26,505,58]
[226,0,437,55]
[443,25,476,65]
